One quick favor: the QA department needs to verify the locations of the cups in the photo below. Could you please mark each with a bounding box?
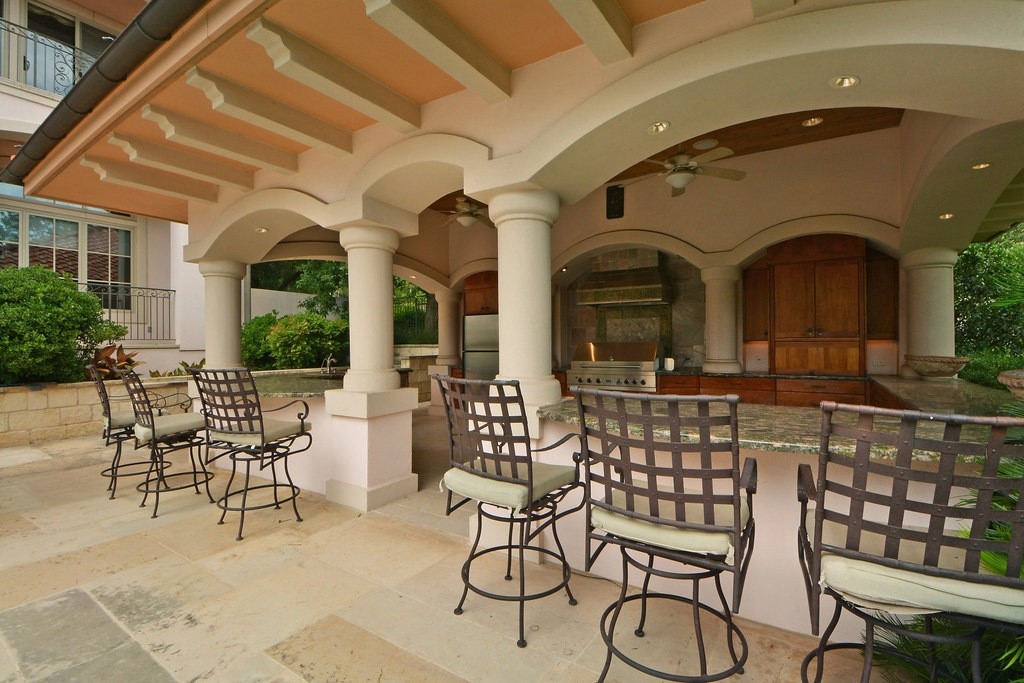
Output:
[664,358,674,372]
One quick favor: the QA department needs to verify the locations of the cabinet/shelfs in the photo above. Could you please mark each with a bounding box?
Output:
[767,259,866,375]
[657,375,871,407]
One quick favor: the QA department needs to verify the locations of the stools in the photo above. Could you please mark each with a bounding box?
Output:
[85,363,169,500]
[184,367,312,541]
[431,374,587,648]
[797,400,1024,683]
[111,366,212,519]
[570,386,758,683]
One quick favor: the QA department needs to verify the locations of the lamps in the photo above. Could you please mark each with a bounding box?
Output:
[455,213,478,228]
[665,171,696,189]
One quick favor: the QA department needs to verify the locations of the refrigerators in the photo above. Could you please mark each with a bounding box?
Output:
[464,315,499,410]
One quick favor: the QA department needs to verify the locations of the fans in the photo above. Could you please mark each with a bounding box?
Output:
[429,197,496,230]
[619,148,747,197]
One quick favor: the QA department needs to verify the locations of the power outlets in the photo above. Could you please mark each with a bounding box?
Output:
[685,355,694,361]
